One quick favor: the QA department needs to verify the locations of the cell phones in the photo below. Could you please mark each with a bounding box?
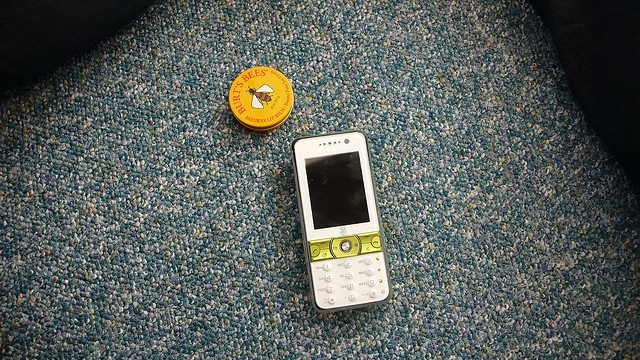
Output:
[291,130,394,315]
